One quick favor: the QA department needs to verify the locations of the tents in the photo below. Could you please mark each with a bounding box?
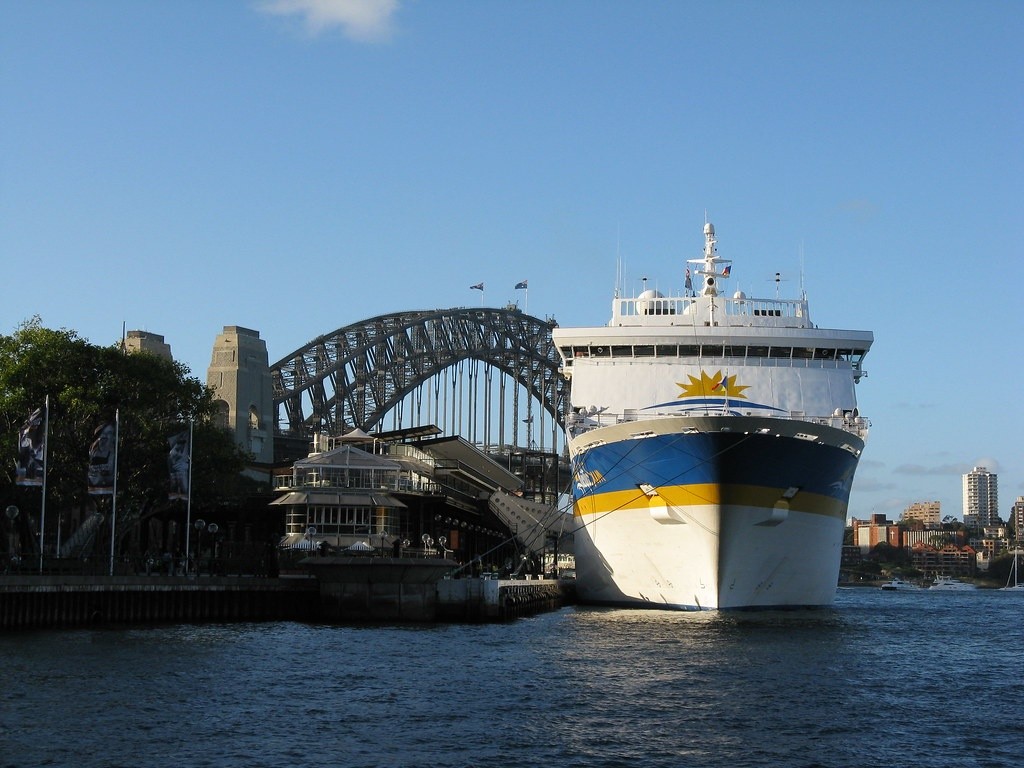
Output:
[332,428,376,456]
[283,537,375,558]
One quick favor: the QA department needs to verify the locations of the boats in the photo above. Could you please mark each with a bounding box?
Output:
[928,575,977,592]
[551,206,874,613]
[881,576,916,590]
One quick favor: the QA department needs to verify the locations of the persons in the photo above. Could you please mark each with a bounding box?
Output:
[392,539,402,558]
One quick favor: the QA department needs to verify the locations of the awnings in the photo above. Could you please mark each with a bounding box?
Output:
[280,535,394,548]
[267,491,407,509]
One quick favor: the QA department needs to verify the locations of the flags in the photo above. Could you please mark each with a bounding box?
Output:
[723,265,730,275]
[712,375,727,391]
[684,268,692,289]
[514,280,526,289]
[469,283,482,290]
